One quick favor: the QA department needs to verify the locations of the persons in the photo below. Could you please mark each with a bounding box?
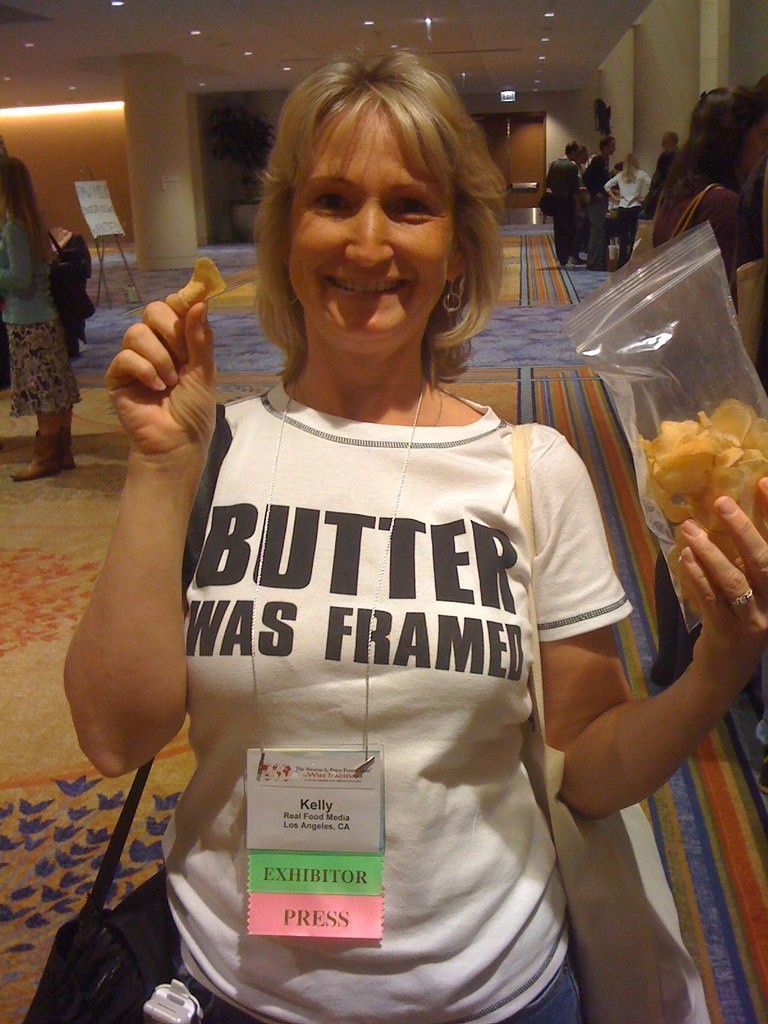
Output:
[652,73,768,393]
[604,153,651,268]
[642,131,678,220]
[0,157,83,480]
[64,39,768,1024]
[545,135,598,266]
[581,135,616,271]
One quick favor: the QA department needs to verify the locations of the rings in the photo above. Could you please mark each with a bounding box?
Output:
[729,588,753,608]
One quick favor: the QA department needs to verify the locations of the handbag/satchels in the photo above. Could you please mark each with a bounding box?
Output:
[24,868,179,1024]
[630,182,668,261]
[538,192,556,217]
[736,160,766,368]
[49,225,96,333]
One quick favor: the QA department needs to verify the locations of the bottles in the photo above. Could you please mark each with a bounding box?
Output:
[127,283,138,304]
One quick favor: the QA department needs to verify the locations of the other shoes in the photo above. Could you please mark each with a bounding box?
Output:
[571,256,586,265]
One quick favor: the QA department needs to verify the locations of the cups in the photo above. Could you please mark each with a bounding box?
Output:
[608,245,616,260]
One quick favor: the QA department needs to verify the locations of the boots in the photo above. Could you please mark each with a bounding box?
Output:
[54,426,77,470]
[9,431,63,480]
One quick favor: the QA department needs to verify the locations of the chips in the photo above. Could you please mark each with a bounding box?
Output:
[177,257,226,310]
[638,400,768,613]
